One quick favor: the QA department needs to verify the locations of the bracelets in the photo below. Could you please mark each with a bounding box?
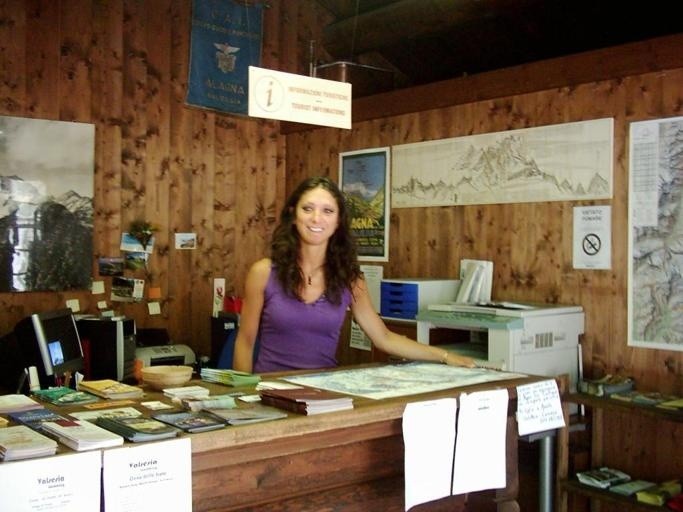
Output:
[442,351,448,364]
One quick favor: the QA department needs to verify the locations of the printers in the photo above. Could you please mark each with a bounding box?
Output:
[417,300,586,418]
[135,328,196,385]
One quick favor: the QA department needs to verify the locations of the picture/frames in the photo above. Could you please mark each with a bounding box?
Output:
[334,143,393,264]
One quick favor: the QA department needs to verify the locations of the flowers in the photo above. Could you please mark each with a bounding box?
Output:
[129,220,162,279]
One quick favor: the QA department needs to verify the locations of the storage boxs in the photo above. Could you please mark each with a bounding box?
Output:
[315,60,394,100]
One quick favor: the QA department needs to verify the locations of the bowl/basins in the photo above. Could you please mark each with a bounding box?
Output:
[142,366,192,390]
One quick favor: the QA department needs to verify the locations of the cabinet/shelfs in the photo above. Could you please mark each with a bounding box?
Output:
[556,372,682,510]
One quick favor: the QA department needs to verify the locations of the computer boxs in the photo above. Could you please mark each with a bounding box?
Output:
[76,317,136,383]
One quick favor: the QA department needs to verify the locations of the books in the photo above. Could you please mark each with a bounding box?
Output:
[453,258,494,304]
[576,465,631,489]
[609,480,657,497]
[1,372,237,461]
[635,481,682,506]
[201,369,354,427]
[610,389,645,402]
[633,391,682,410]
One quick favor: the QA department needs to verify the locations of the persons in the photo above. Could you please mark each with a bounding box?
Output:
[231,177,480,376]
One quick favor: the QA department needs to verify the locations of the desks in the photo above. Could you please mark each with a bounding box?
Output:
[0,356,557,510]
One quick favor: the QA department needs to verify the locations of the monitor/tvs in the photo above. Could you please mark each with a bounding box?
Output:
[0,308,85,394]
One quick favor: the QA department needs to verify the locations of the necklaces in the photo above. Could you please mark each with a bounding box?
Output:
[299,264,323,286]
